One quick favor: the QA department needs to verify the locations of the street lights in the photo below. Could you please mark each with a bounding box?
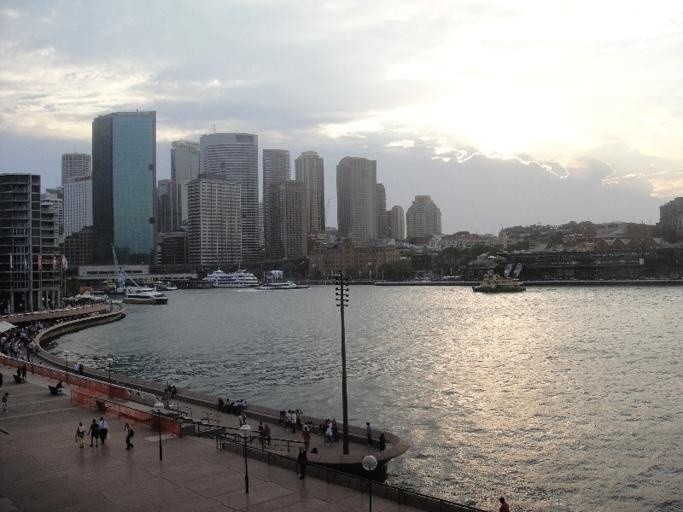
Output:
[361,454,378,512]
[239,423,252,493]
[105,358,113,382]
[153,401,165,460]
[63,349,70,384]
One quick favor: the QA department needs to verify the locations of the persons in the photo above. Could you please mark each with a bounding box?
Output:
[259,422,263,431]
[366,422,372,448]
[124,423,134,449]
[499,496,509,512]
[56,381,65,395]
[99,417,108,445]
[279,407,337,451]
[263,424,272,446]
[297,446,307,480]
[2,393,10,413]
[0,300,89,387]
[163,384,177,400]
[86,419,99,447]
[379,433,386,451]
[217,397,248,427]
[75,421,87,448]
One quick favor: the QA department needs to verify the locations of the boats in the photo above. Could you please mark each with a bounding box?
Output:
[472,273,526,292]
[255,279,310,290]
[122,284,168,304]
[198,266,259,288]
